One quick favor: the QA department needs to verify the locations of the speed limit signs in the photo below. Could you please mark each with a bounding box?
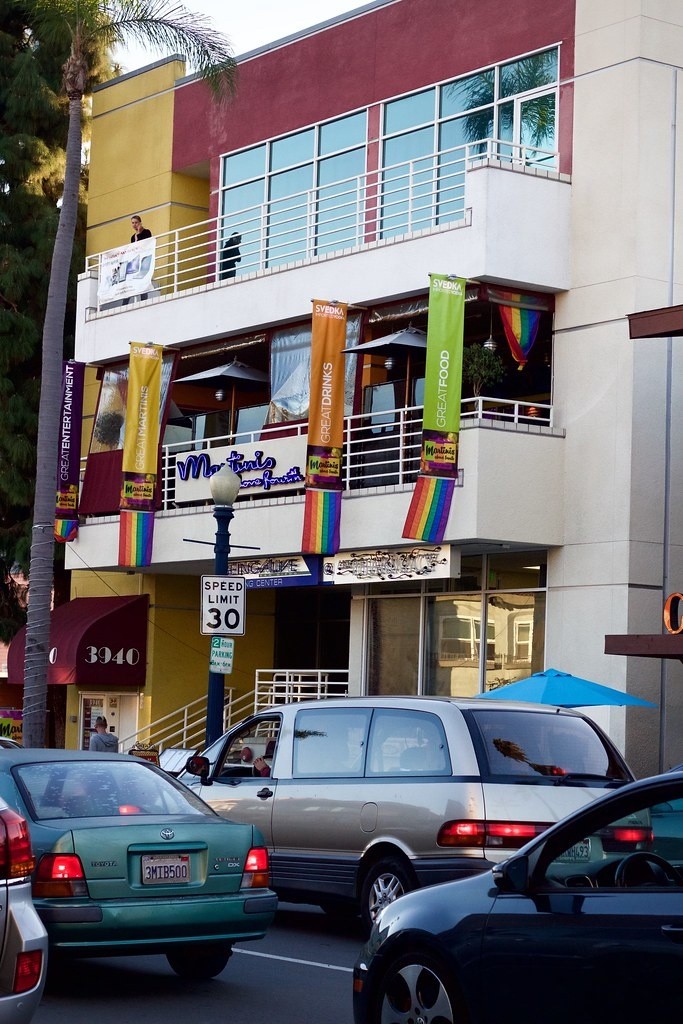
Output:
[202,575,245,635]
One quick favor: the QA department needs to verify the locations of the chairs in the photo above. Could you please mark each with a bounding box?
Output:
[398,748,426,773]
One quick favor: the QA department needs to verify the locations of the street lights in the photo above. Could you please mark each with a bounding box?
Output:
[206,465,242,746]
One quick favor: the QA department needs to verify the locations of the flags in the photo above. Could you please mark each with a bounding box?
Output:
[495,289,540,370]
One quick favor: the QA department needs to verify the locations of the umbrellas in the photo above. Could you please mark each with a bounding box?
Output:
[473,668,660,708]
[339,322,427,482]
[171,355,268,445]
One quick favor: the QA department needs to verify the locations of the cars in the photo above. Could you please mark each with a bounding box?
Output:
[0,746,279,980]
[351,772,683,1024]
[0,797,50,1024]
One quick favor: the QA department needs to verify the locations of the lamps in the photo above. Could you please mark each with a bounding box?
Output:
[484,302,498,353]
[216,389,226,402]
[384,327,396,371]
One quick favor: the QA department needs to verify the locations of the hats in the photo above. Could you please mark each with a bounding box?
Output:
[95,716,108,727]
[260,741,276,759]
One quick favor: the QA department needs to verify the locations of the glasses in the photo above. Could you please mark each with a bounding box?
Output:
[94,724,99,728]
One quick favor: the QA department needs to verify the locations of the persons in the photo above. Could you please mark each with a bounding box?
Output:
[122,215,152,305]
[89,716,119,752]
[222,232,241,280]
[253,755,271,777]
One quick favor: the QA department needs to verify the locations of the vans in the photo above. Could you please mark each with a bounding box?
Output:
[157,696,652,940]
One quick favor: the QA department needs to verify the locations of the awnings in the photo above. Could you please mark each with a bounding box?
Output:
[7,594,149,686]
